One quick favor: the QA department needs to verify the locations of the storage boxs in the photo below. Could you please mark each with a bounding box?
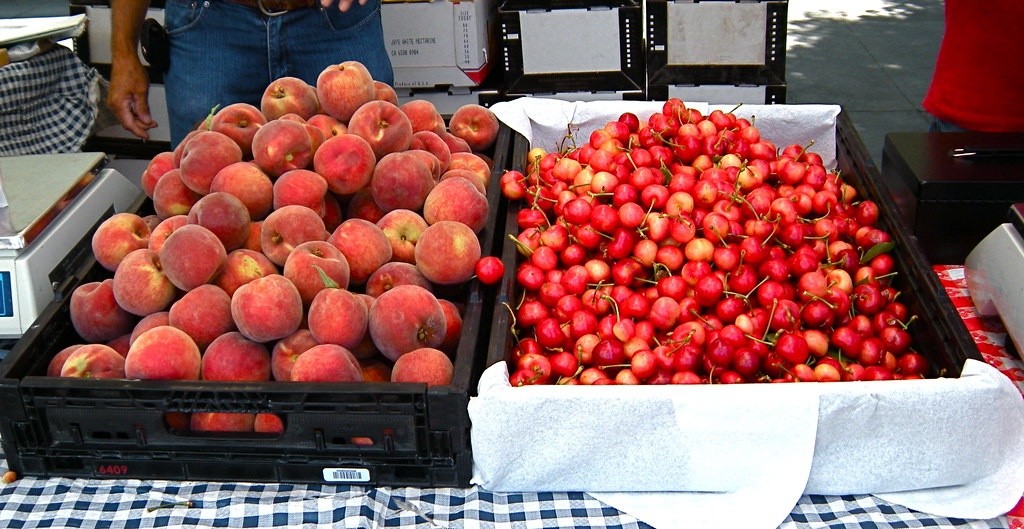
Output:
[492,0,644,95]
[379,0,492,90]
[482,96,989,489]
[0,113,513,490]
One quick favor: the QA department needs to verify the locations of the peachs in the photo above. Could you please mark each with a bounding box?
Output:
[48,61,498,444]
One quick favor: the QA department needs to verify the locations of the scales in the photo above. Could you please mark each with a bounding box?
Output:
[0,152,142,340]
[963,203,1024,359]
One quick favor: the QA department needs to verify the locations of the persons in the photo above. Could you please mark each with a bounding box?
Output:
[920,0,1024,134]
[105,0,394,152]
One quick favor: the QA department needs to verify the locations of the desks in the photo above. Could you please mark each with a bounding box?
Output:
[879,123,1023,265]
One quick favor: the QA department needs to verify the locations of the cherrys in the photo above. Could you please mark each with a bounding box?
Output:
[470,97,927,385]
